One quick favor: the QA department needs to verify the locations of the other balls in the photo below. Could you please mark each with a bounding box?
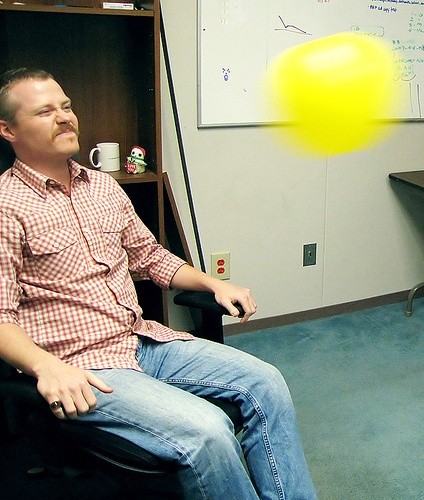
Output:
[263,32,410,157]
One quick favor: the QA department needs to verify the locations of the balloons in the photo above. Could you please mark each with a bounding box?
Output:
[273,31,408,156]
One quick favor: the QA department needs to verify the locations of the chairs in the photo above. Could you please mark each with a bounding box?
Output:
[0,139,248,500]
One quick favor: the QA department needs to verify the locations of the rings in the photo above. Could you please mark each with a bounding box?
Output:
[50,401,61,410]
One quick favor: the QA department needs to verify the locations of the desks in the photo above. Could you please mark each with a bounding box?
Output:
[389,171,424,315]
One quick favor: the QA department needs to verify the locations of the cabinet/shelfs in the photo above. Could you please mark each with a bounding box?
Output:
[0,0,168,350]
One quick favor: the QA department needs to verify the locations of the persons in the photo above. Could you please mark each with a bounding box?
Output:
[0,67,321,500]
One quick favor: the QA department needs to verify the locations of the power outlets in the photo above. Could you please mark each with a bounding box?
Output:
[211,253,230,280]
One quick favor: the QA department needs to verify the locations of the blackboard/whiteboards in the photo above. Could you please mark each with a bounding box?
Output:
[195,0,424,129]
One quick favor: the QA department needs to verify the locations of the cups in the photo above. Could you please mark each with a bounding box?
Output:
[89,142,121,172]
